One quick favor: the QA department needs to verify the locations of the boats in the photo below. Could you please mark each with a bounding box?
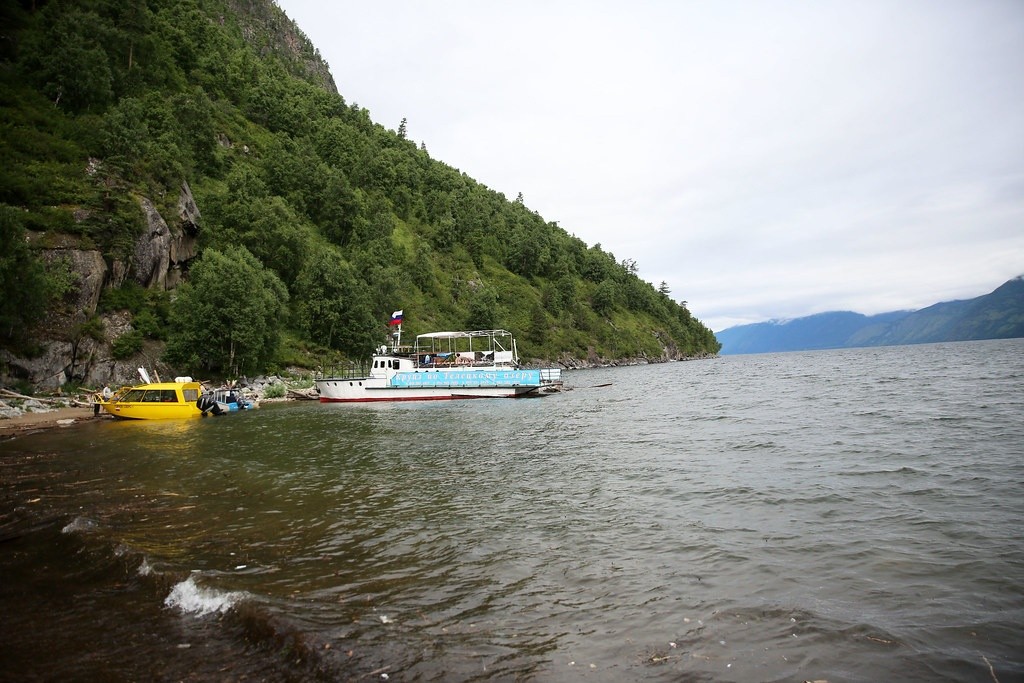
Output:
[91,381,210,420]
[314,318,565,403]
[192,382,257,417]
[286,387,319,401]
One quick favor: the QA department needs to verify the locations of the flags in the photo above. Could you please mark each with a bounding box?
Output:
[389,310,403,326]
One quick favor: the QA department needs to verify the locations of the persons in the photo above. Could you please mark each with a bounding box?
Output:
[92,382,126,417]
